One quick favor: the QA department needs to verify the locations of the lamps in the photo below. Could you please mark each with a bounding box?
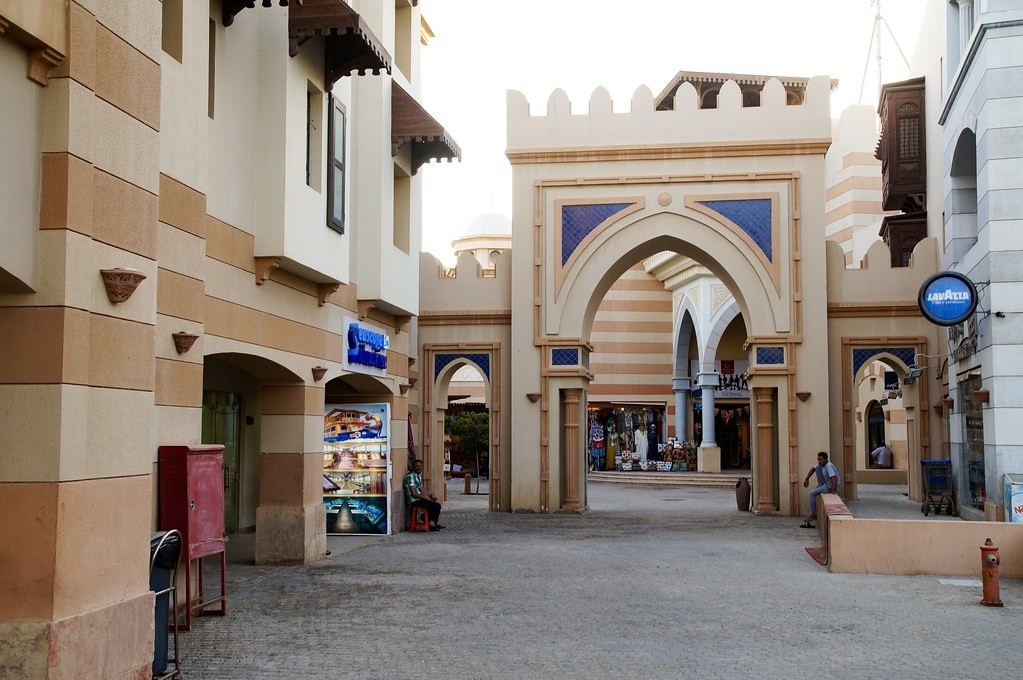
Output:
[310,367,329,381]
[100,270,146,303]
[879,353,948,405]
[399,358,417,395]
[172,332,199,354]
[527,393,541,402]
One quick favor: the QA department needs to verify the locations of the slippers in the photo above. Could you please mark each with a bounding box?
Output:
[800,522,815,528]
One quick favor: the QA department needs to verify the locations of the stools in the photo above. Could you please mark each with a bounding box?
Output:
[410,506,430,533]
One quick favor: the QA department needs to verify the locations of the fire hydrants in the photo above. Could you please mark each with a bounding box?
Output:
[979,537,1004,606]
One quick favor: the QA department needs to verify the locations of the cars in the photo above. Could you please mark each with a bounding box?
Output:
[443,460,463,475]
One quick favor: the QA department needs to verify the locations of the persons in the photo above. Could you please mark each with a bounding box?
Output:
[870,442,892,470]
[606,423,659,471]
[803,452,841,523]
[404,460,446,532]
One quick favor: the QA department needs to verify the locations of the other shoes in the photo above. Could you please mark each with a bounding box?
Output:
[430,525,439,531]
[437,524,446,529]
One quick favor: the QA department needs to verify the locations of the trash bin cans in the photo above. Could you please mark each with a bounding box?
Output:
[735,477,751,511]
[150,531,179,674]
[1002,472,1023,522]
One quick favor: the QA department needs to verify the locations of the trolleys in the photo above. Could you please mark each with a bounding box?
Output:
[152,528,186,680]
[968,460,986,511]
[918,459,957,517]
